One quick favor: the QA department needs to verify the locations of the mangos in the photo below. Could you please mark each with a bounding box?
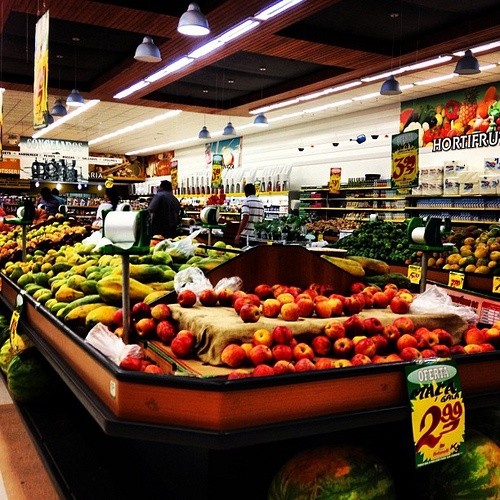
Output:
[0,222,86,261]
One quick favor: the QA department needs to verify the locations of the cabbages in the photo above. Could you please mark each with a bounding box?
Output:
[334,219,418,263]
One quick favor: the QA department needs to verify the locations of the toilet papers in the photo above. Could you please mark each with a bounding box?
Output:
[412,157,500,195]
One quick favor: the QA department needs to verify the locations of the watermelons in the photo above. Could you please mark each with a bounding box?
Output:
[399,107,414,133]
[265,428,500,500]
[0,315,77,404]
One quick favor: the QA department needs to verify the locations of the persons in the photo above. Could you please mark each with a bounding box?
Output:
[96,185,120,220]
[146,180,181,248]
[0,200,24,217]
[234,183,265,250]
[36,187,68,216]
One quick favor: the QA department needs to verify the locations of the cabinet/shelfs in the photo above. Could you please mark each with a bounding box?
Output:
[302,188,500,246]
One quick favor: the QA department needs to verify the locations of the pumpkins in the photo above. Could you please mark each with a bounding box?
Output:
[406,228,500,274]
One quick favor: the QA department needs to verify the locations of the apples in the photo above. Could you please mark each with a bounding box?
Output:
[111,280,500,377]
[4,239,390,329]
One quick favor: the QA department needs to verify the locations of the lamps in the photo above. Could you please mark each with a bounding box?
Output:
[177,3,211,36]
[47,113,54,124]
[51,55,67,116]
[199,126,210,138]
[134,35,161,62]
[452,48,480,75]
[66,37,84,106]
[380,75,402,95]
[253,112,268,126]
[222,121,237,136]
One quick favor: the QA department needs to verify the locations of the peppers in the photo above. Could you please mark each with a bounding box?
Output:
[423,99,462,141]
[487,99,500,117]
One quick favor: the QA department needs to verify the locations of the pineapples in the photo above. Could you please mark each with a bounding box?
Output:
[459,86,477,126]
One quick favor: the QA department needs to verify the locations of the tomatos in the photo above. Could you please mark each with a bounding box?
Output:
[472,86,500,132]
